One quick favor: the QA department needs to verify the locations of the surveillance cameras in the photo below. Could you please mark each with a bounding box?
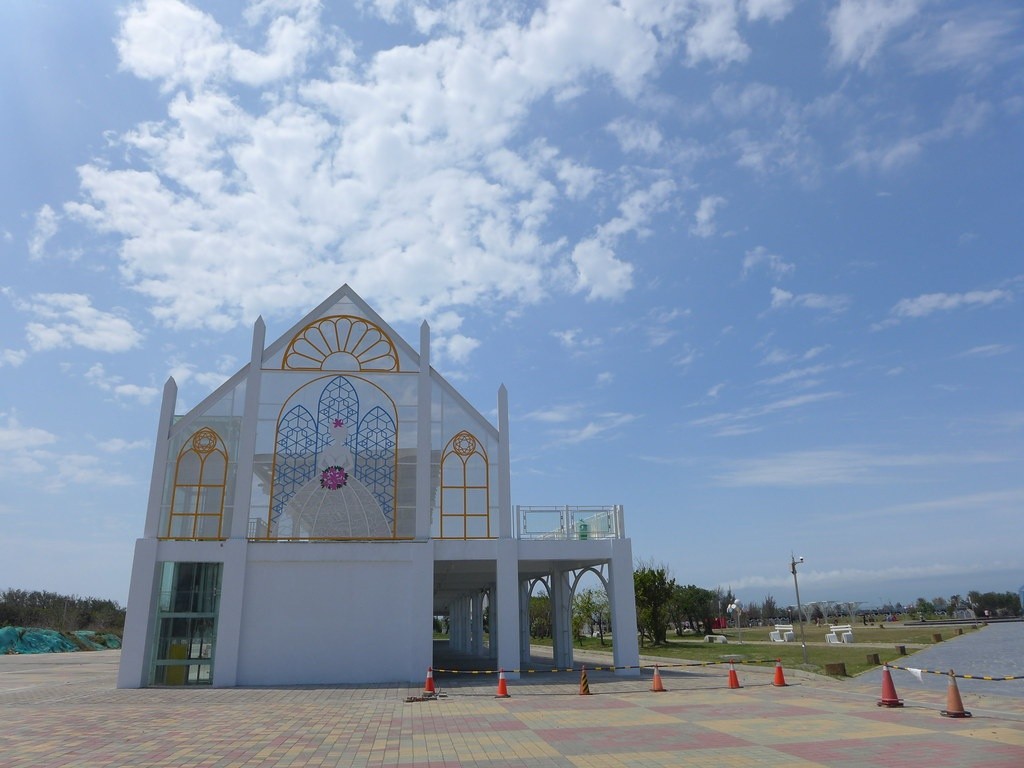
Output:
[800,557,804,561]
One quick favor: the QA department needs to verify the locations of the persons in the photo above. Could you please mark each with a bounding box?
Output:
[919,610,924,621]
[863,615,866,625]
[984,608,989,620]
[675,623,689,634]
[814,615,838,626]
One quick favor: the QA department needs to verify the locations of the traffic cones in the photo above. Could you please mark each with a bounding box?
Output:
[648,663,667,692]
[878,661,903,707]
[726,659,743,689]
[577,665,594,695]
[772,657,789,687]
[940,668,973,718]
[423,666,436,696]
[495,666,511,698]
